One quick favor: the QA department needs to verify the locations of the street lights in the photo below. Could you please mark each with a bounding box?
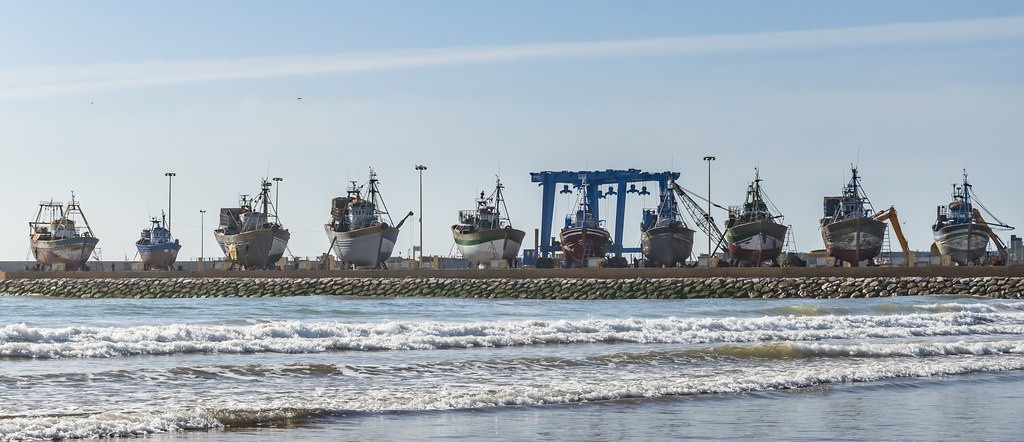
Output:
[272,177,283,224]
[164,172,176,238]
[199,208,207,261]
[703,156,716,267]
[414,163,428,268]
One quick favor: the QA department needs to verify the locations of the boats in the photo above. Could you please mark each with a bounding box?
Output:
[451,161,527,266]
[28,190,100,271]
[640,157,696,268]
[212,161,291,269]
[559,174,612,266]
[709,165,788,267]
[324,165,400,268]
[818,147,888,266]
[135,208,182,270]
[931,169,1015,267]
